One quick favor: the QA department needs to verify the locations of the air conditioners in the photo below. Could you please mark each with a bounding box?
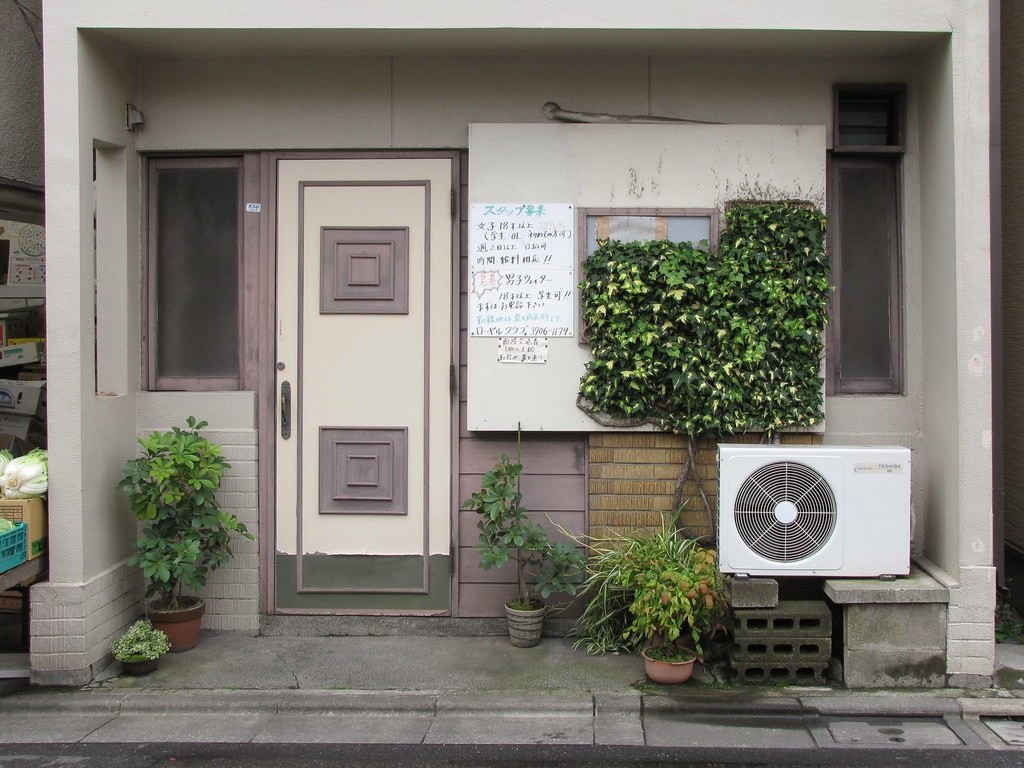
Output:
[717,442,912,580]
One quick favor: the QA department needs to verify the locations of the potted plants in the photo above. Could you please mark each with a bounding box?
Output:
[110,620,171,676]
[627,543,734,686]
[116,416,255,652]
[460,453,589,648]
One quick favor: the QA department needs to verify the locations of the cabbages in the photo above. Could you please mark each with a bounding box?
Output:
[0,448,61,534]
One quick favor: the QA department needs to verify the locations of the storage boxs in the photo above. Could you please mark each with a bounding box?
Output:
[0,415,46,458]
[0,495,47,575]
[0,379,47,420]
[0,320,46,381]
